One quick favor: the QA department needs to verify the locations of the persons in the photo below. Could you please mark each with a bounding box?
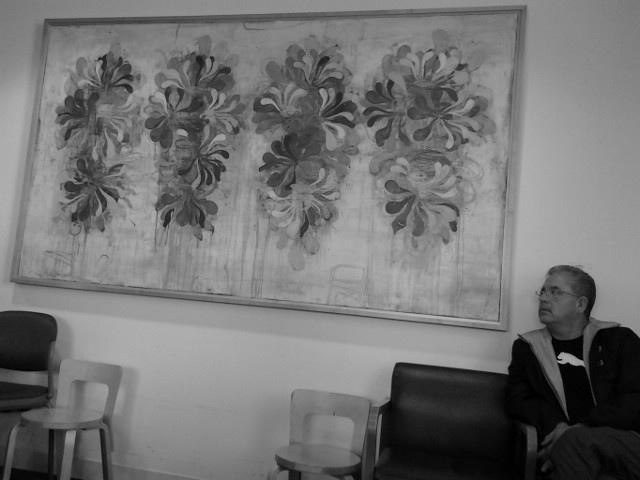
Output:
[499,265,640,480]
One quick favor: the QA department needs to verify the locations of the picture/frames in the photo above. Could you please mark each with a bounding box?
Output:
[8,2,529,333]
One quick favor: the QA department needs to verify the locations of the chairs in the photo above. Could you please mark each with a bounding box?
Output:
[1,309,124,480]
[268,362,538,479]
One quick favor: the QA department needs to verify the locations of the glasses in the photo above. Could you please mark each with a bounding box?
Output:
[535,288,582,299]
